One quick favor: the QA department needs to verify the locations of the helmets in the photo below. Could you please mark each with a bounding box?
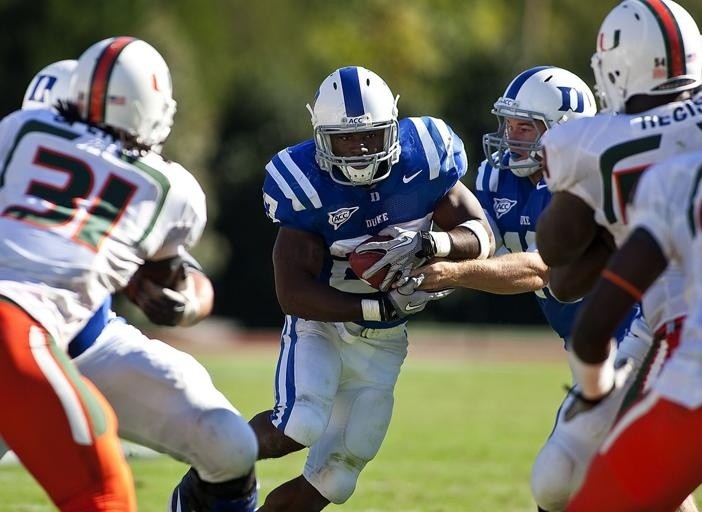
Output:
[312,65,400,166]
[20,58,81,111]
[63,34,174,147]
[494,66,598,165]
[590,1,702,114]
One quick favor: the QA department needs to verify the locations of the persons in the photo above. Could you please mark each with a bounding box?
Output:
[391,62,649,510]
[528,1,702,511]
[0,59,261,509]
[165,65,505,511]
[560,144,702,511]
[0,33,209,510]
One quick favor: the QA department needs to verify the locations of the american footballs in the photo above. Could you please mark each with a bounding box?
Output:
[348,236,404,291]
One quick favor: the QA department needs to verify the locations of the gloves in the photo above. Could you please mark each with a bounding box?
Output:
[387,273,457,319]
[133,278,187,330]
[355,222,437,293]
